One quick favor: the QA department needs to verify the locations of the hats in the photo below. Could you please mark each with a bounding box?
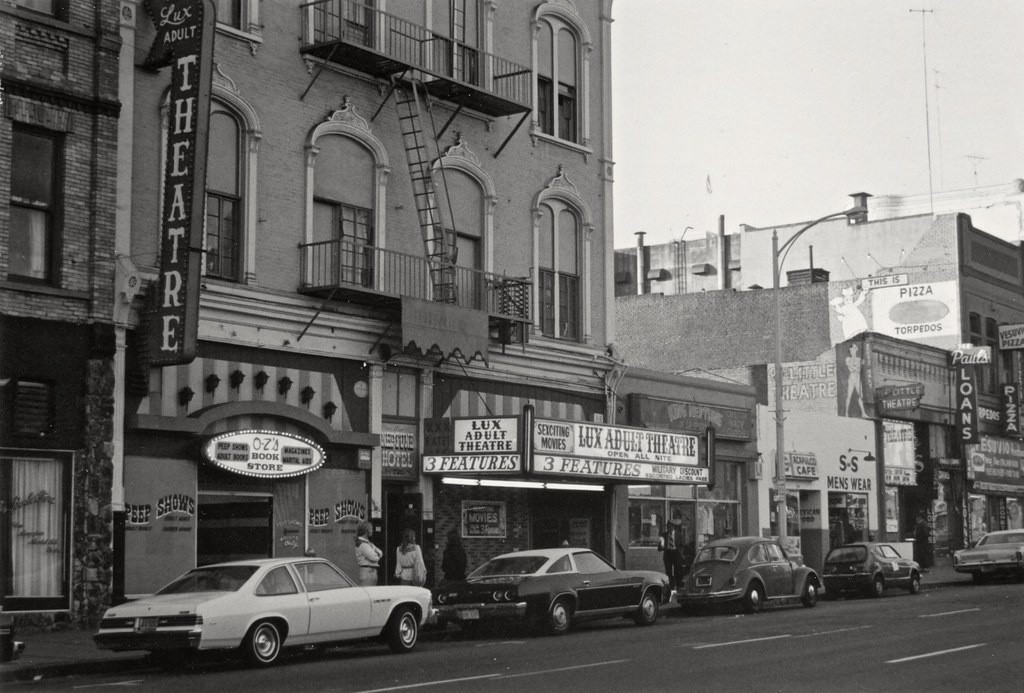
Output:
[357,520,371,535]
[916,509,928,522]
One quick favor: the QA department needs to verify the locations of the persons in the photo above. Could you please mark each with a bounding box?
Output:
[657,520,682,591]
[394,529,427,588]
[440,531,466,581]
[913,511,930,572]
[353,520,384,586]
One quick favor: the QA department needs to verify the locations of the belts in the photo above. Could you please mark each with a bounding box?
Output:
[362,565,376,568]
[401,566,413,569]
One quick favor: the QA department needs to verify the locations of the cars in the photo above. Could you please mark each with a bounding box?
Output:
[93,557,432,667]
[431,547,673,636]
[676,537,822,614]
[823,542,921,599]
[952,529,1024,584]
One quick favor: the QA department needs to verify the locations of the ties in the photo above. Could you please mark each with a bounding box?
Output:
[669,533,674,550]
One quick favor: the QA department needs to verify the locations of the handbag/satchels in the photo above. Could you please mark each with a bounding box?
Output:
[413,545,426,587]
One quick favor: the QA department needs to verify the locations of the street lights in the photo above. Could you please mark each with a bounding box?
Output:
[771,192,874,537]
